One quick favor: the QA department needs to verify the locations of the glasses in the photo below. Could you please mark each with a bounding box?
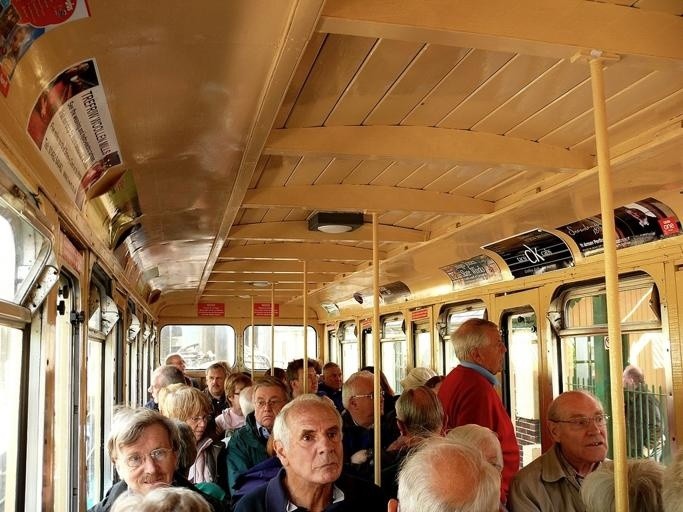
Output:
[171,362,187,366]
[353,391,385,399]
[547,414,611,427]
[182,414,212,422]
[112,446,175,468]
[233,393,239,395]
[255,400,283,407]
[300,373,321,380]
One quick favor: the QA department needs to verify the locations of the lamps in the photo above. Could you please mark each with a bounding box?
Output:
[308,213,365,235]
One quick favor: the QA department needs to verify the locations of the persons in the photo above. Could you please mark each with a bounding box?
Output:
[110,486,212,512]
[437,318,521,512]
[214,372,254,437]
[40,62,90,117]
[623,365,666,463]
[338,370,394,485]
[370,386,449,489]
[264,367,288,388]
[79,158,115,194]
[582,456,665,512]
[166,354,200,390]
[425,375,447,396]
[226,377,289,499]
[320,362,345,413]
[286,359,320,399]
[400,367,437,392]
[387,432,500,512]
[85,407,229,512]
[164,386,224,501]
[234,393,391,512]
[445,424,509,512]
[507,391,612,512]
[201,364,229,415]
[624,207,662,236]
[143,366,186,413]
